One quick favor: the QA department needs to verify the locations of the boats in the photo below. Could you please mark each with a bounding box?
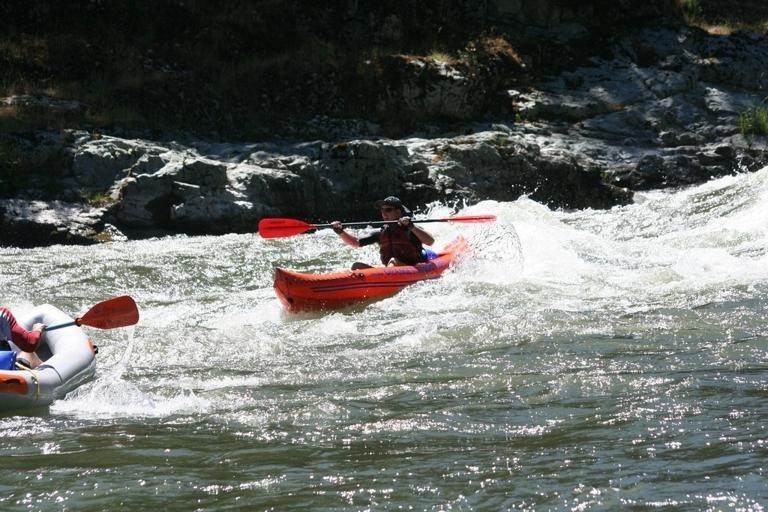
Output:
[272,234,465,317]
[1,300,99,405]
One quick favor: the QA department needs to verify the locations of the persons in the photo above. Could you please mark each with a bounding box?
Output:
[331,195,435,270]
[0,306,47,370]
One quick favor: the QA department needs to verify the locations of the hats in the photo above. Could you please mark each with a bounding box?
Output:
[374,196,402,209]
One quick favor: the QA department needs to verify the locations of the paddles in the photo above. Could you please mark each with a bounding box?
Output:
[42,296,139,330]
[259,214,496,239]
